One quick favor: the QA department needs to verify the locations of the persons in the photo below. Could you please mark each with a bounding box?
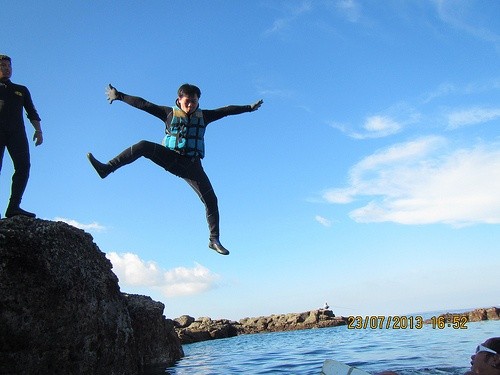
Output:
[88,84,264,255]
[471,337,500,375]
[0,54,42,217]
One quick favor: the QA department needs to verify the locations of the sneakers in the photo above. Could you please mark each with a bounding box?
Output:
[5,208,36,218]
[88,153,111,179]
[209,241,229,255]
